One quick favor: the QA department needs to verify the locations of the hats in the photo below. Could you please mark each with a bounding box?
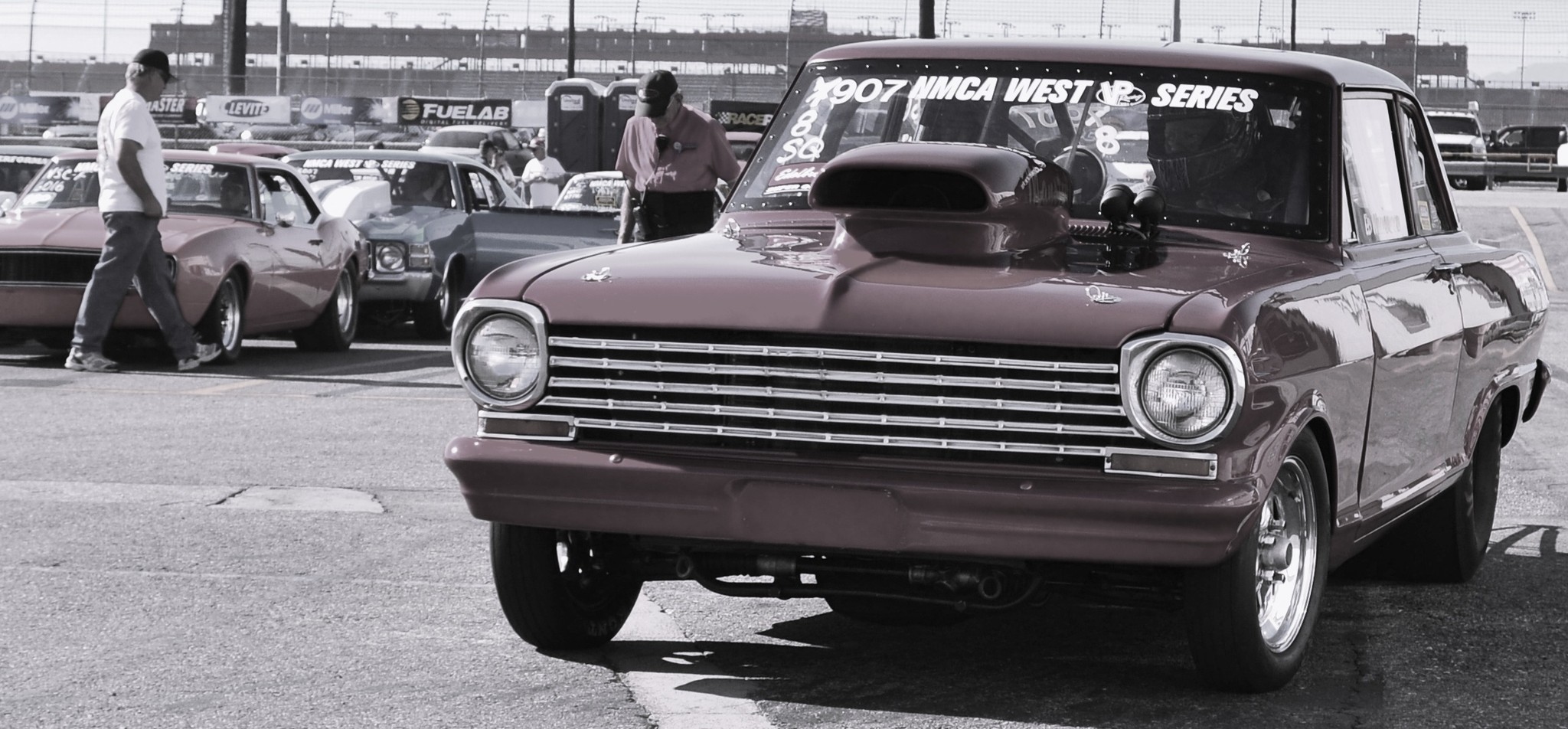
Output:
[529,138,545,149]
[634,70,678,119]
[132,48,179,84]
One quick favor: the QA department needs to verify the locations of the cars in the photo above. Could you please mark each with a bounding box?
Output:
[1095,130,1157,195]
[0,97,767,367]
[441,36,1557,692]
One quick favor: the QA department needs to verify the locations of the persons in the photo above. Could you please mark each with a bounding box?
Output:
[60,47,224,374]
[522,138,567,210]
[213,175,249,215]
[475,139,516,211]
[1134,82,1279,223]
[416,168,446,203]
[615,70,741,243]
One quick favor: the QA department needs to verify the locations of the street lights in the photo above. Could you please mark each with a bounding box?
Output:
[383,10,399,30]
[1211,24,1229,42]
[434,11,668,31]
[940,18,961,36]
[698,12,715,37]
[1432,28,1447,104]
[1375,27,1389,59]
[1104,23,1123,38]
[996,21,1019,37]
[1266,26,1282,47]
[1321,27,1336,52]
[1514,11,1537,89]
[888,15,904,37]
[333,10,353,27]
[1052,23,1067,38]
[720,13,744,37]
[1157,23,1172,40]
[856,14,880,35]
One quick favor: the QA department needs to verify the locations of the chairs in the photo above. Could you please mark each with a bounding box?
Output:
[1259,126,1311,224]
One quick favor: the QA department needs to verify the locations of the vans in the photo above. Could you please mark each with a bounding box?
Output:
[1483,122,1568,184]
[1427,111,1490,192]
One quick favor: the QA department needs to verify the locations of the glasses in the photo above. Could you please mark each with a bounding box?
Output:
[159,72,170,83]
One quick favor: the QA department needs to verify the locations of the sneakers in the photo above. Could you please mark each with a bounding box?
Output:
[64,348,120,373]
[178,342,222,372]
